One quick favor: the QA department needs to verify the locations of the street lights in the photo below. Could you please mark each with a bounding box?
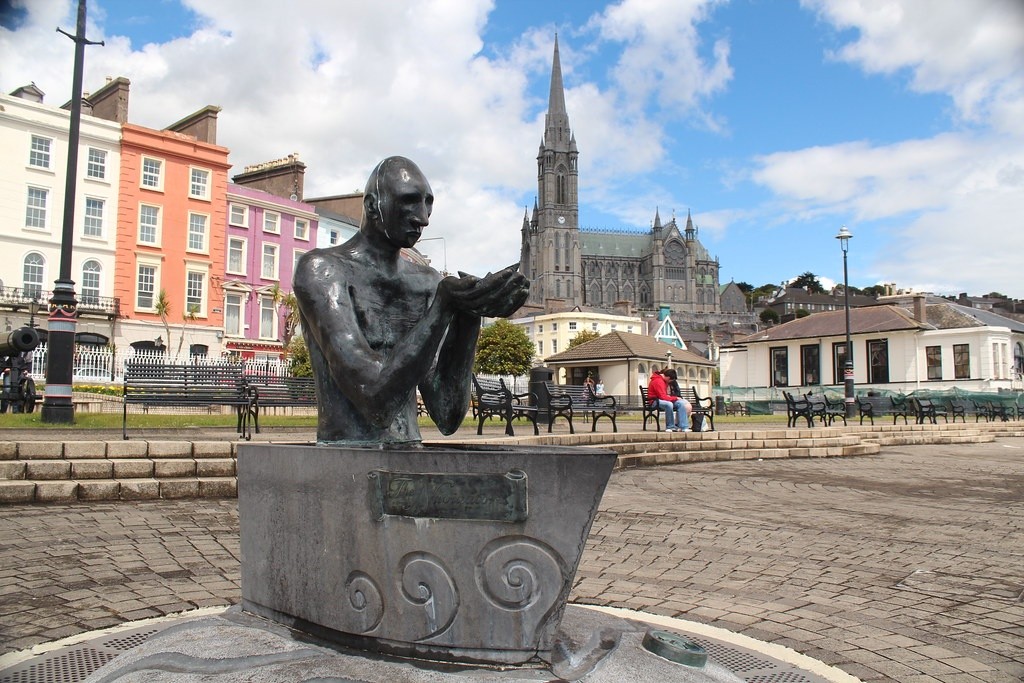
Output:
[419,237,448,278]
[836,226,856,418]
[28,296,40,329]
[732,323,758,332]
[750,288,755,312]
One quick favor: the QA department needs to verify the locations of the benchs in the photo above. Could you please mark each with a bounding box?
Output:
[856,395,908,427]
[949,400,989,423]
[723,400,751,417]
[529,379,617,435]
[639,385,716,432]
[123,359,317,441]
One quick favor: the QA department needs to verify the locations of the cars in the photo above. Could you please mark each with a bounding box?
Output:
[71,366,124,383]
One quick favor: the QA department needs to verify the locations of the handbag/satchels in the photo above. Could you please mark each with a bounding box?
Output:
[691,413,709,432]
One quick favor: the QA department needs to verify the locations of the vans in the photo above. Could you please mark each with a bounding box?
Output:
[218,369,277,398]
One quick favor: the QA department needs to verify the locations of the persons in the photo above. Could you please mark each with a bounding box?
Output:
[596,379,605,396]
[293,156,531,444]
[583,377,595,399]
[647,368,693,432]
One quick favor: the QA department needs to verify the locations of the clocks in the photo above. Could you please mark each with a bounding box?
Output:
[557,216,565,224]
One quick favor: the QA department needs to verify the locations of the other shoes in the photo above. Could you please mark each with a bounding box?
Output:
[665,428,672,432]
[677,427,692,432]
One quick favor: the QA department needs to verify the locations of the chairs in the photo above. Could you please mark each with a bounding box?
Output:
[471,394,503,422]
[417,402,430,417]
[987,400,1024,422]
[471,373,540,436]
[910,397,948,424]
[781,390,847,427]
